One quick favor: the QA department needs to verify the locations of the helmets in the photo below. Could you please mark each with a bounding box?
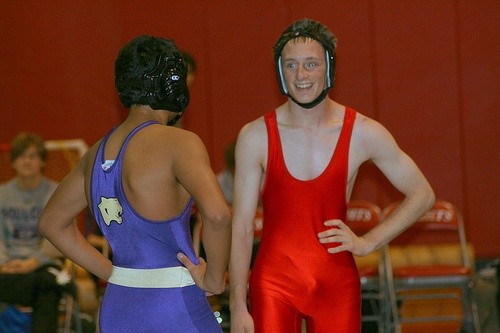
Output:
[273,18,337,95]
[115,34,190,113]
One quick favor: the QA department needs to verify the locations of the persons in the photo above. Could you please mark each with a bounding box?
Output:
[193,140,235,257]
[230,19,436,333]
[0,133,74,333]
[162,52,195,128]
[38,34,233,333]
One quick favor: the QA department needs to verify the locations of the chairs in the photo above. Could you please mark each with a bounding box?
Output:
[15,256,83,333]
[196,206,264,332]
[383,200,483,332]
[345,199,394,332]
